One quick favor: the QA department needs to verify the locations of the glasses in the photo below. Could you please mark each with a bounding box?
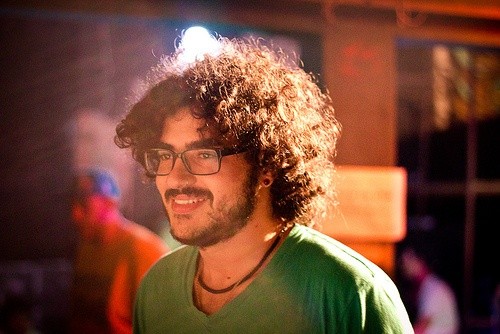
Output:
[143,144,253,176]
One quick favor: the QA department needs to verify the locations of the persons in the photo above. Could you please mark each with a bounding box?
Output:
[46,164,172,334]
[112,40,418,334]
[400,247,460,334]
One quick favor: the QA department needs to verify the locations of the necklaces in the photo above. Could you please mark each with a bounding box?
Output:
[196,217,291,295]
